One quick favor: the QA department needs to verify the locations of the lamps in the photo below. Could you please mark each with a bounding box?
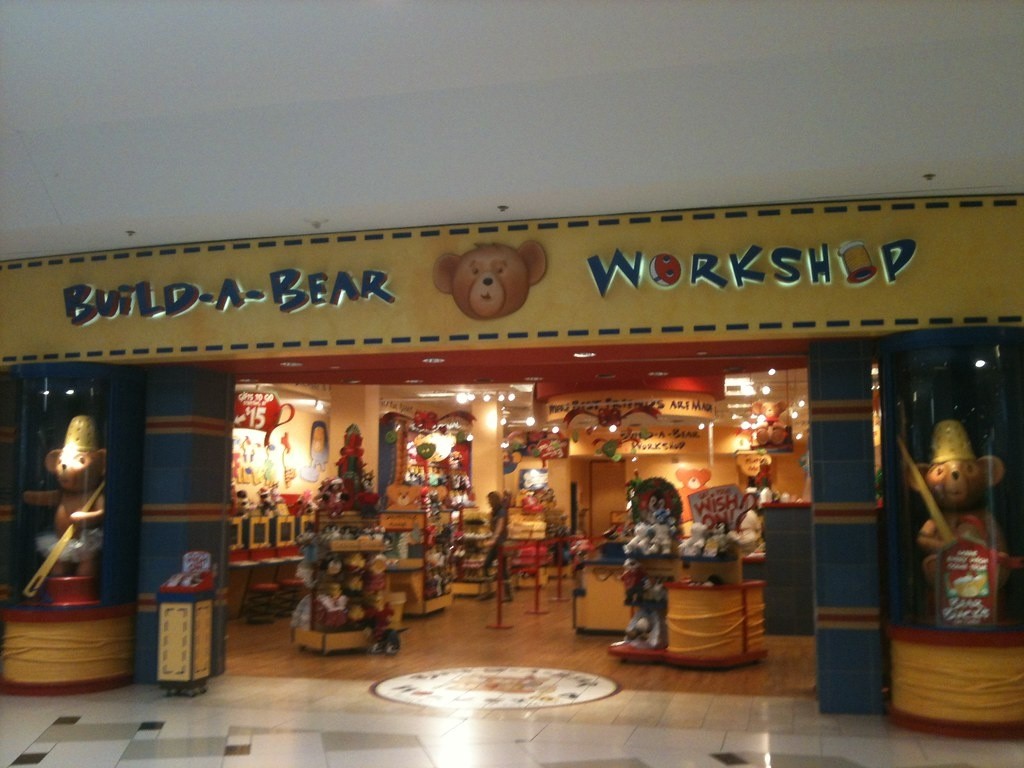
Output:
[724,376,756,396]
[418,393,455,397]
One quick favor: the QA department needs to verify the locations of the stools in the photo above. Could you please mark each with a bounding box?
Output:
[275,577,303,616]
[237,581,277,624]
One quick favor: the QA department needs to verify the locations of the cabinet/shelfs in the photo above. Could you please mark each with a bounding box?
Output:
[378,503,556,618]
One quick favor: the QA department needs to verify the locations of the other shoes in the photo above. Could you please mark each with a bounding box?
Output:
[478,590,495,601]
[503,589,513,602]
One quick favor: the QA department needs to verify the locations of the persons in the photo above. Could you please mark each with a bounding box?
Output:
[475,491,514,603]
